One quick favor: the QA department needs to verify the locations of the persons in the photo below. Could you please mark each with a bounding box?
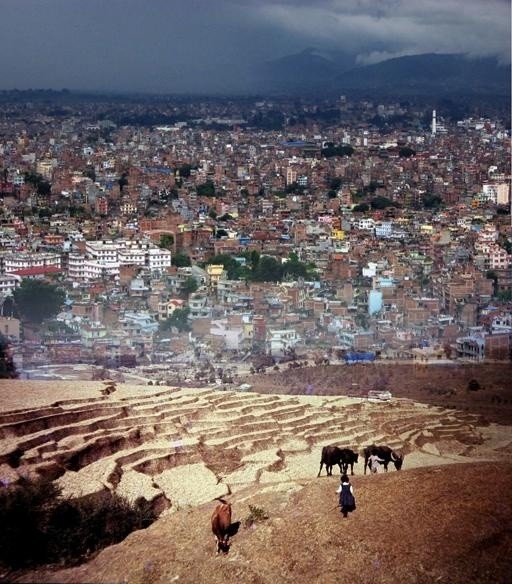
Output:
[335,474,356,520]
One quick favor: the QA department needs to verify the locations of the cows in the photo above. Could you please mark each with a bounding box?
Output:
[210,502,232,556]
[316,444,359,478]
[363,444,404,475]
